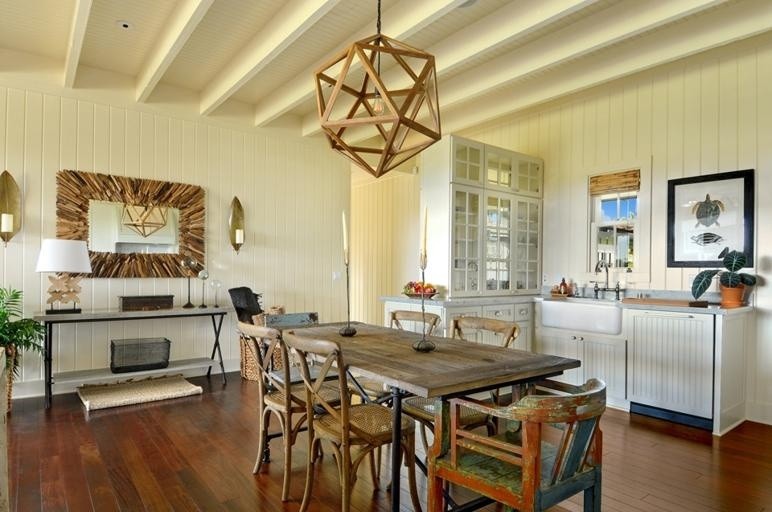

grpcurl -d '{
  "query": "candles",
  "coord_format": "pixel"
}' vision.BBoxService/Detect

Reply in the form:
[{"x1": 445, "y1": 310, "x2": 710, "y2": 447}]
[
  {"x1": 341, "y1": 210, "x2": 349, "y2": 264},
  {"x1": 420, "y1": 202, "x2": 428, "y2": 264}
]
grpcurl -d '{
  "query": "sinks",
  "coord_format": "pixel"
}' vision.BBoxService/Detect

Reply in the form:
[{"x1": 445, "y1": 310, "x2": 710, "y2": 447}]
[{"x1": 533, "y1": 295, "x2": 621, "y2": 336}]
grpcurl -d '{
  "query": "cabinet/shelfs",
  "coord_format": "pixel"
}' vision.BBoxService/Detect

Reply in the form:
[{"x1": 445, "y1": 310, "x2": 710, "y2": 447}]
[
  {"x1": 384, "y1": 303, "x2": 514, "y2": 400},
  {"x1": 515, "y1": 301, "x2": 533, "y2": 351},
  {"x1": 540, "y1": 328, "x2": 628, "y2": 411}
]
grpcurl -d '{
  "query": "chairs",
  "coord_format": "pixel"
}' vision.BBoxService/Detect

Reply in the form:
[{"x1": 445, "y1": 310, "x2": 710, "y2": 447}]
[
  {"x1": 428, "y1": 375, "x2": 607, "y2": 512},
  {"x1": 280, "y1": 327, "x2": 424, "y2": 512},
  {"x1": 340, "y1": 311, "x2": 439, "y2": 478},
  {"x1": 234, "y1": 321, "x2": 352, "y2": 512},
  {"x1": 384, "y1": 318, "x2": 519, "y2": 511}
]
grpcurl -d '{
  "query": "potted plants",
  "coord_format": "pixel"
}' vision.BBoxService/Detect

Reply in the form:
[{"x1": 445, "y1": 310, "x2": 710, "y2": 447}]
[
  {"x1": 691, "y1": 247, "x2": 757, "y2": 308},
  {"x1": 0, "y1": 288, "x2": 52, "y2": 415}
]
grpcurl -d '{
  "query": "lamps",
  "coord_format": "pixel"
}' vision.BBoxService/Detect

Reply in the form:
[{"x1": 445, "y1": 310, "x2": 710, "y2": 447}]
[
  {"x1": 0, "y1": 170, "x2": 22, "y2": 243},
  {"x1": 311, "y1": 0, "x2": 443, "y2": 179},
  {"x1": 34, "y1": 237, "x2": 94, "y2": 314},
  {"x1": 228, "y1": 196, "x2": 244, "y2": 251}
]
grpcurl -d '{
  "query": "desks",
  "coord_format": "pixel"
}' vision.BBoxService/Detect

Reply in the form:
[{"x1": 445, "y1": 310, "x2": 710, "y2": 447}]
[{"x1": 34, "y1": 301, "x2": 235, "y2": 409}]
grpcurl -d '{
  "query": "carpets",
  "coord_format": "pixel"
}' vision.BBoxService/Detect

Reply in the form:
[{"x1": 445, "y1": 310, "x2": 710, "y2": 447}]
[{"x1": 75, "y1": 374, "x2": 203, "y2": 413}]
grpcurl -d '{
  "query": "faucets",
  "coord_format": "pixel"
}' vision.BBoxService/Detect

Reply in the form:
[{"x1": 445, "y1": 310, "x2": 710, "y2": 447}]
[{"x1": 594, "y1": 258, "x2": 609, "y2": 289}]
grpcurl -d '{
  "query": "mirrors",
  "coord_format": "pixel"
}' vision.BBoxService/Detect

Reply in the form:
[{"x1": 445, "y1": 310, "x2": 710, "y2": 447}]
[{"x1": 53, "y1": 167, "x2": 208, "y2": 277}]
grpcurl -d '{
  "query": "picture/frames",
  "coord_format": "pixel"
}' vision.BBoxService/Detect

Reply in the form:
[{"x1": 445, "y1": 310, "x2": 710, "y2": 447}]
[{"x1": 667, "y1": 168, "x2": 754, "y2": 268}]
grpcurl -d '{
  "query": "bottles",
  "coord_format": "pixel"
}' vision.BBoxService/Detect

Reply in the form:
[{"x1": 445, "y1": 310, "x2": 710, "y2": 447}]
[
  {"x1": 553, "y1": 285, "x2": 560, "y2": 293},
  {"x1": 559, "y1": 277, "x2": 567, "y2": 294}
]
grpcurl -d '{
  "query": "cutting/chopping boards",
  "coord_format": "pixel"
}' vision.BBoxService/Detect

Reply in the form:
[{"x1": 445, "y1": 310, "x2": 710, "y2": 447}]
[{"x1": 623, "y1": 297, "x2": 709, "y2": 307}]
